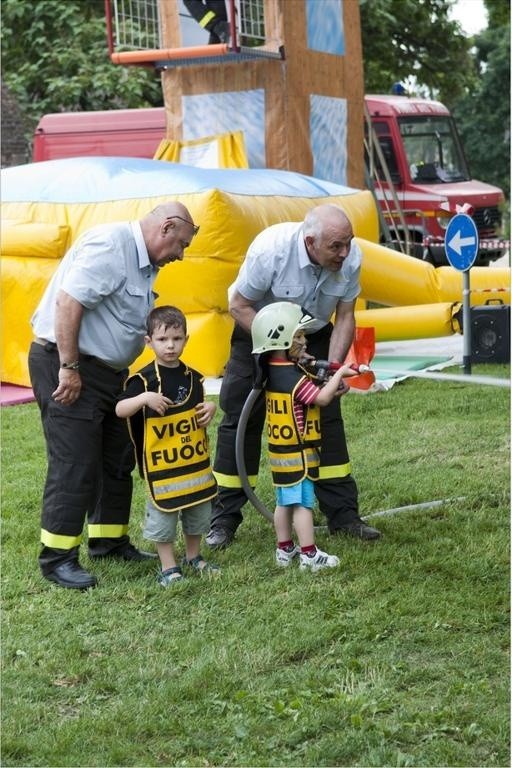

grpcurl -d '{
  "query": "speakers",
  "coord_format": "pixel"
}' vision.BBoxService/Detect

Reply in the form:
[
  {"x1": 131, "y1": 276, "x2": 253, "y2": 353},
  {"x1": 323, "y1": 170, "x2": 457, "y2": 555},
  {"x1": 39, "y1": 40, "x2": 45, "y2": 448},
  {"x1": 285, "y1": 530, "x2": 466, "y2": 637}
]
[{"x1": 470, "y1": 304, "x2": 510, "y2": 363}]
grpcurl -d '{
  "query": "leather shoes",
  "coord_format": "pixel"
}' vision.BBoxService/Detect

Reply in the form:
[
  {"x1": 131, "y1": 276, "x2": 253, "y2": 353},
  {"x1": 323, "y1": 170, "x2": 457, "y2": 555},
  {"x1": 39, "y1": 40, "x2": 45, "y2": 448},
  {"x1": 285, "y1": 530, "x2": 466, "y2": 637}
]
[
  {"x1": 43, "y1": 557, "x2": 96, "y2": 589},
  {"x1": 205, "y1": 521, "x2": 237, "y2": 548},
  {"x1": 327, "y1": 518, "x2": 381, "y2": 541},
  {"x1": 88, "y1": 540, "x2": 160, "y2": 563}
]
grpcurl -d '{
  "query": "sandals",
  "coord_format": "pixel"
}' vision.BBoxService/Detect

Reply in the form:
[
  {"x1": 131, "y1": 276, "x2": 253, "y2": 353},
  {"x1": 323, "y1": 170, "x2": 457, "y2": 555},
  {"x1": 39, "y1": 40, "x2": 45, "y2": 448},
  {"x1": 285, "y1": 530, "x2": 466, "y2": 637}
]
[
  {"x1": 156, "y1": 566, "x2": 188, "y2": 594},
  {"x1": 183, "y1": 555, "x2": 222, "y2": 582}
]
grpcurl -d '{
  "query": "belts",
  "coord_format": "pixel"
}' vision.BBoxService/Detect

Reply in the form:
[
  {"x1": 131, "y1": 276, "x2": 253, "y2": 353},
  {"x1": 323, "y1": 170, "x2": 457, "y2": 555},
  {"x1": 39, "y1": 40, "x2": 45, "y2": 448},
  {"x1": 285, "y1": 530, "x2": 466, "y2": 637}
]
[{"x1": 32, "y1": 336, "x2": 120, "y2": 376}]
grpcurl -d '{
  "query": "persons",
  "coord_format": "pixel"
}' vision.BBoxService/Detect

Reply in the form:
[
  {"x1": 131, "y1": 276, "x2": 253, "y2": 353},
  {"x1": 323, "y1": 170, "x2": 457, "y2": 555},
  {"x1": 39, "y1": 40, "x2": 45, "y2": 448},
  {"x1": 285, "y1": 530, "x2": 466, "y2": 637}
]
[
  {"x1": 28, "y1": 198, "x2": 206, "y2": 590},
  {"x1": 182, "y1": 0, "x2": 234, "y2": 45},
  {"x1": 248, "y1": 301, "x2": 361, "y2": 572},
  {"x1": 111, "y1": 305, "x2": 222, "y2": 589},
  {"x1": 199, "y1": 201, "x2": 384, "y2": 548}
]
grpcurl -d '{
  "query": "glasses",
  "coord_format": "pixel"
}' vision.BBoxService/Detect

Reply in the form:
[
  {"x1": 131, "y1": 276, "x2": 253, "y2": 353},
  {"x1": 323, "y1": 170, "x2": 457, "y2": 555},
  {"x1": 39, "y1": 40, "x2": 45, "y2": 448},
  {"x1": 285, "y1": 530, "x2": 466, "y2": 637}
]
[{"x1": 166, "y1": 214, "x2": 200, "y2": 234}]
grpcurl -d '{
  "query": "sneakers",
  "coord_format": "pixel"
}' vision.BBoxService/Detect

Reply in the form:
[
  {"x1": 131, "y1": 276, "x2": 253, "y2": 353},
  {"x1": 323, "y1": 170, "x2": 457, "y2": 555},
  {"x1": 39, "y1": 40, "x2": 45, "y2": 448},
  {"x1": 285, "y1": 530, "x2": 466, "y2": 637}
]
[
  {"x1": 274, "y1": 540, "x2": 301, "y2": 567},
  {"x1": 296, "y1": 545, "x2": 340, "y2": 572}
]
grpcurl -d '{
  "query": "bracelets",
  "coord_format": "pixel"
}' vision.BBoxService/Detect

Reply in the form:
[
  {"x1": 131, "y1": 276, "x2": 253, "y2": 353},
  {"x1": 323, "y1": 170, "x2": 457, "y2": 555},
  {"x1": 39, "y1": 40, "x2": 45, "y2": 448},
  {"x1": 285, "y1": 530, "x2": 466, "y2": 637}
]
[{"x1": 59, "y1": 361, "x2": 80, "y2": 370}]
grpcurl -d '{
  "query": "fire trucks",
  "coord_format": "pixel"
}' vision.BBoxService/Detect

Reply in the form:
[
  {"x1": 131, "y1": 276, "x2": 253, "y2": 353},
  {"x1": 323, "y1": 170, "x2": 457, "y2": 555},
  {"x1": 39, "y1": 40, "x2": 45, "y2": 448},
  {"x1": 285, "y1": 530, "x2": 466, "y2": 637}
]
[{"x1": 33, "y1": 85, "x2": 505, "y2": 270}]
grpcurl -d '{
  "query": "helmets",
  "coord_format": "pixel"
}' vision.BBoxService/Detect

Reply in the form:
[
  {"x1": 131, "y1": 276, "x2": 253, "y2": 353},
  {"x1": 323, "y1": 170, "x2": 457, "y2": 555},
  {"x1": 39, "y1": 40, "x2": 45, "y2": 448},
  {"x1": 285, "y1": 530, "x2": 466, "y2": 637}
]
[{"x1": 246, "y1": 300, "x2": 316, "y2": 356}]
